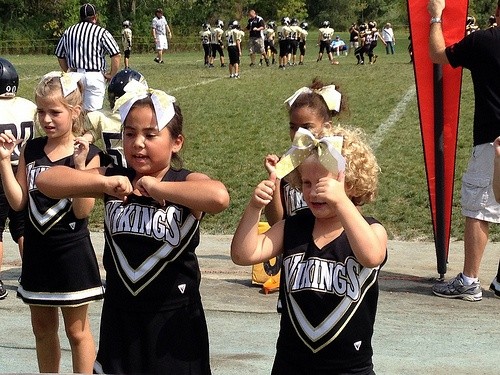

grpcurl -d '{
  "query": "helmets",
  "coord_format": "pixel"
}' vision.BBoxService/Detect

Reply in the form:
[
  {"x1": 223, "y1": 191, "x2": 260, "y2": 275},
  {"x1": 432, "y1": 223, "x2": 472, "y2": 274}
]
[
  {"x1": 107, "y1": 69, "x2": 148, "y2": 110},
  {"x1": 227, "y1": 20, "x2": 241, "y2": 30},
  {"x1": 215, "y1": 19, "x2": 226, "y2": 29},
  {"x1": 122, "y1": 20, "x2": 133, "y2": 28},
  {"x1": 357, "y1": 19, "x2": 378, "y2": 31},
  {"x1": 202, "y1": 23, "x2": 211, "y2": 31},
  {"x1": 281, "y1": 16, "x2": 309, "y2": 31},
  {"x1": 322, "y1": 20, "x2": 331, "y2": 28},
  {"x1": 0, "y1": 57, "x2": 19, "y2": 97},
  {"x1": 267, "y1": 20, "x2": 276, "y2": 28}
]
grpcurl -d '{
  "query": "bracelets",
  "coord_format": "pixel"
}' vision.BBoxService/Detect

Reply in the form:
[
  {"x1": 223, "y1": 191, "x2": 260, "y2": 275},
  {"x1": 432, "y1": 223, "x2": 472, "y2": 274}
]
[{"x1": 430, "y1": 16, "x2": 441, "y2": 23}]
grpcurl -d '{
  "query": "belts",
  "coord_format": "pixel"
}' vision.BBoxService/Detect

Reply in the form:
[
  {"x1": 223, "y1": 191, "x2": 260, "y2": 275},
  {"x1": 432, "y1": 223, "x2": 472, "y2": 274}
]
[{"x1": 70, "y1": 69, "x2": 100, "y2": 72}]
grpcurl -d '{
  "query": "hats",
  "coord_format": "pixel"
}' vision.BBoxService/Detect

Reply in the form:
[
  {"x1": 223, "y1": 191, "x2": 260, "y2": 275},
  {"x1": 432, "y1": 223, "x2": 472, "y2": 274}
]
[{"x1": 80, "y1": 3, "x2": 97, "y2": 17}]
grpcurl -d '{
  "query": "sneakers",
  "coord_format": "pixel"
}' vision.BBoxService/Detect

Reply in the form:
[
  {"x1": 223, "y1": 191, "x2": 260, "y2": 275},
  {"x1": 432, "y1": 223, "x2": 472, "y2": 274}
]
[{"x1": 431, "y1": 273, "x2": 483, "y2": 301}]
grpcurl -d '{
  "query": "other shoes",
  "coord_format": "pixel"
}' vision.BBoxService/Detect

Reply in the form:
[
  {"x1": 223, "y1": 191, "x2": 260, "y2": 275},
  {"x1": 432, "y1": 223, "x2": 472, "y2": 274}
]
[
  {"x1": 204, "y1": 62, "x2": 227, "y2": 68},
  {"x1": 279, "y1": 65, "x2": 286, "y2": 70},
  {"x1": 356, "y1": 60, "x2": 365, "y2": 65},
  {"x1": 287, "y1": 62, "x2": 297, "y2": 66},
  {"x1": 230, "y1": 74, "x2": 241, "y2": 79},
  {"x1": 368, "y1": 55, "x2": 378, "y2": 64},
  {"x1": 299, "y1": 61, "x2": 303, "y2": 65},
  {"x1": 249, "y1": 59, "x2": 276, "y2": 67}
]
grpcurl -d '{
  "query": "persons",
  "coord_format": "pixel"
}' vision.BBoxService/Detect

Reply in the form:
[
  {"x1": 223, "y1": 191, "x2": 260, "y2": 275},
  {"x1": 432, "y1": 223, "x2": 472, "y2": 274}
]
[
  {"x1": 0, "y1": 72, "x2": 103, "y2": 375},
  {"x1": 35, "y1": 80, "x2": 230, "y2": 375},
  {"x1": 0, "y1": 58, "x2": 38, "y2": 298},
  {"x1": 264, "y1": 79, "x2": 348, "y2": 227},
  {"x1": 243, "y1": 10, "x2": 270, "y2": 67},
  {"x1": 121, "y1": 20, "x2": 132, "y2": 69},
  {"x1": 317, "y1": 21, "x2": 412, "y2": 65},
  {"x1": 152, "y1": 9, "x2": 172, "y2": 64},
  {"x1": 84, "y1": 69, "x2": 148, "y2": 284},
  {"x1": 200, "y1": 20, "x2": 244, "y2": 79},
  {"x1": 231, "y1": 125, "x2": 388, "y2": 375},
  {"x1": 55, "y1": 3, "x2": 121, "y2": 112},
  {"x1": 428, "y1": 0, "x2": 500, "y2": 301},
  {"x1": 259, "y1": 17, "x2": 308, "y2": 69}
]
